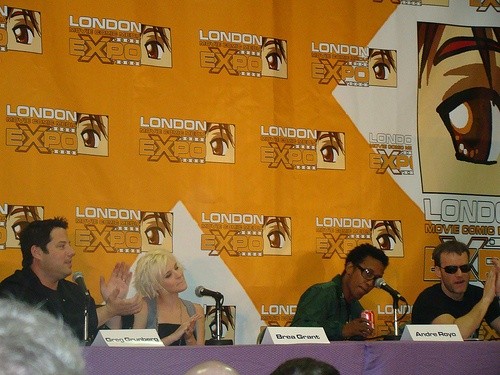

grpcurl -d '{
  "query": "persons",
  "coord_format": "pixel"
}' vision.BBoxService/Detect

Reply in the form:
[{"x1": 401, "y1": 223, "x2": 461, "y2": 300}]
[
  {"x1": 182, "y1": 360, "x2": 241, "y2": 375},
  {"x1": 411, "y1": 241, "x2": 500, "y2": 341},
  {"x1": 0, "y1": 292, "x2": 89, "y2": 374},
  {"x1": 290, "y1": 243, "x2": 390, "y2": 342},
  {"x1": 268, "y1": 358, "x2": 341, "y2": 375},
  {"x1": 0, "y1": 215, "x2": 145, "y2": 348},
  {"x1": 129, "y1": 249, "x2": 207, "y2": 348}
]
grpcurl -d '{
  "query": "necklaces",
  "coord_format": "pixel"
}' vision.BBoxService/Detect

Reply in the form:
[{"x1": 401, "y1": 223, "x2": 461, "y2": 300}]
[{"x1": 156, "y1": 302, "x2": 181, "y2": 333}]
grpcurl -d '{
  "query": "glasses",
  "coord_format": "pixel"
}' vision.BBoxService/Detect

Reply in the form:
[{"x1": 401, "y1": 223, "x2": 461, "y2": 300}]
[
  {"x1": 353, "y1": 262, "x2": 382, "y2": 281},
  {"x1": 439, "y1": 264, "x2": 471, "y2": 274}
]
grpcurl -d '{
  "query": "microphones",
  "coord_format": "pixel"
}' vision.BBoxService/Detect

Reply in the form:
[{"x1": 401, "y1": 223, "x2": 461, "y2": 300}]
[
  {"x1": 195, "y1": 285, "x2": 223, "y2": 300},
  {"x1": 72, "y1": 271, "x2": 91, "y2": 304},
  {"x1": 375, "y1": 278, "x2": 406, "y2": 303}
]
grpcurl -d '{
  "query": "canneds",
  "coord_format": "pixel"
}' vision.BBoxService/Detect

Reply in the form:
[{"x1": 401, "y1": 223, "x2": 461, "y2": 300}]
[{"x1": 361, "y1": 310, "x2": 375, "y2": 331}]
